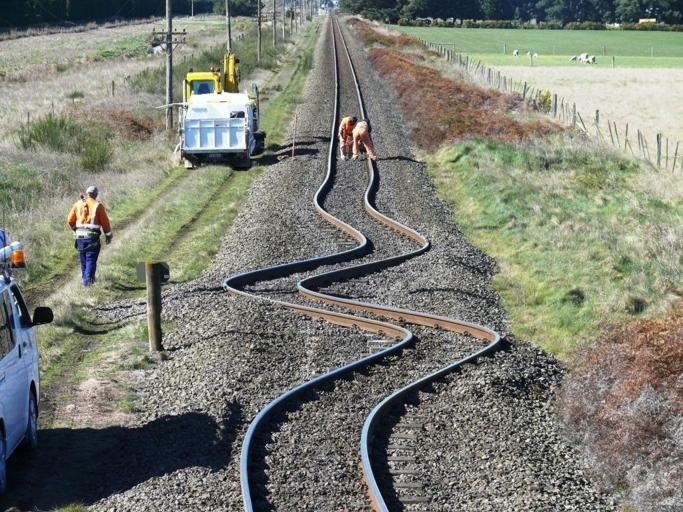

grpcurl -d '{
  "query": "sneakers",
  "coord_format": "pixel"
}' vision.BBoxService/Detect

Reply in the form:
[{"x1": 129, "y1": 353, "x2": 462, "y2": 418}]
[{"x1": 342, "y1": 152, "x2": 379, "y2": 161}]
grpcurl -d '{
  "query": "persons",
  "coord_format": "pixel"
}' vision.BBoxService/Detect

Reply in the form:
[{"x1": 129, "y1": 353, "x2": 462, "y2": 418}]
[
  {"x1": 352, "y1": 119, "x2": 376, "y2": 162},
  {"x1": 337, "y1": 116, "x2": 358, "y2": 160},
  {"x1": 67, "y1": 186, "x2": 112, "y2": 286}
]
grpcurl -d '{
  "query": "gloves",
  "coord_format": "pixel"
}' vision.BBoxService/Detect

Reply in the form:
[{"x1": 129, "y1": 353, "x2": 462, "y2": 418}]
[{"x1": 106, "y1": 236, "x2": 111, "y2": 245}]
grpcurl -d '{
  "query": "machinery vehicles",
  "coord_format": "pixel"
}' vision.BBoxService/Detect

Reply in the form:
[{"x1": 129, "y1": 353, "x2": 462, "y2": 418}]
[{"x1": 174, "y1": 49, "x2": 267, "y2": 174}]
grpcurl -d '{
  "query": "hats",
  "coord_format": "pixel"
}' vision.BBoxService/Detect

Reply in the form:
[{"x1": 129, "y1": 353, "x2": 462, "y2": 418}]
[{"x1": 86, "y1": 185, "x2": 97, "y2": 196}]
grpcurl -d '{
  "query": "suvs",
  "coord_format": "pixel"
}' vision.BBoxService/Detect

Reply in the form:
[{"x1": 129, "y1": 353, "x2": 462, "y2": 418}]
[{"x1": 0, "y1": 226, "x2": 59, "y2": 509}]
